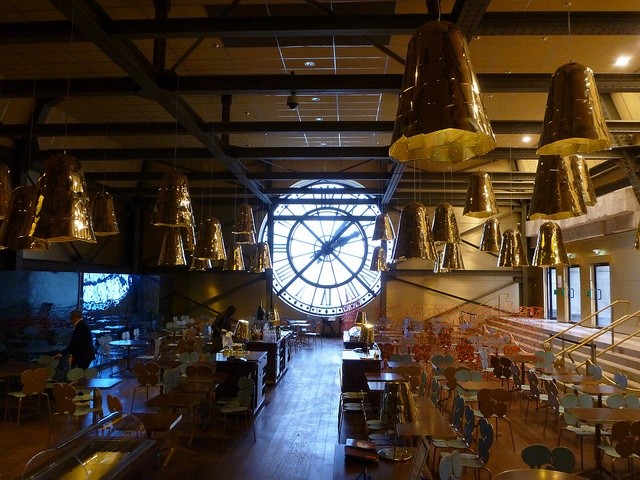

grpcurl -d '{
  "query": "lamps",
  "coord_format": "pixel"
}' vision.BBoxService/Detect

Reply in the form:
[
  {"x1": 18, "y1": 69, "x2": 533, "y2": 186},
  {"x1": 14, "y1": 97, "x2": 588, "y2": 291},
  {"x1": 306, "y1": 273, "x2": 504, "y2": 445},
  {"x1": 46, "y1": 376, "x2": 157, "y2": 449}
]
[
  {"x1": 532, "y1": 215, "x2": 569, "y2": 267},
  {"x1": 18, "y1": 81, "x2": 97, "y2": 243},
  {"x1": 496, "y1": 135, "x2": 530, "y2": 268},
  {"x1": 188, "y1": 190, "x2": 213, "y2": 268},
  {"x1": 433, "y1": 243, "x2": 452, "y2": 273},
  {"x1": 150, "y1": 82, "x2": 195, "y2": 227},
  {"x1": 576, "y1": 156, "x2": 598, "y2": 205},
  {"x1": 157, "y1": 229, "x2": 187, "y2": 266},
  {"x1": 480, "y1": 157, "x2": 502, "y2": 252},
  {"x1": 223, "y1": 237, "x2": 245, "y2": 270},
  {"x1": 535, "y1": 3, "x2": 612, "y2": 156},
  {"x1": 392, "y1": 161, "x2": 432, "y2": 261},
  {"x1": 251, "y1": 199, "x2": 272, "y2": 270},
  {"x1": 378, "y1": 382, "x2": 417, "y2": 462},
  {"x1": 231, "y1": 161, "x2": 257, "y2": 236},
  {"x1": 286, "y1": 93, "x2": 299, "y2": 109},
  {"x1": 463, "y1": 156, "x2": 500, "y2": 217},
  {"x1": 0, "y1": 159, "x2": 13, "y2": 220},
  {"x1": 269, "y1": 308, "x2": 279, "y2": 328},
  {"x1": 358, "y1": 323, "x2": 375, "y2": 360},
  {"x1": 369, "y1": 181, "x2": 387, "y2": 272},
  {"x1": 354, "y1": 311, "x2": 367, "y2": 326},
  {"x1": 91, "y1": 133, "x2": 119, "y2": 236},
  {"x1": 432, "y1": 163, "x2": 460, "y2": 243},
  {"x1": 388, "y1": 2, "x2": 497, "y2": 164},
  {"x1": 527, "y1": 156, "x2": 587, "y2": 219},
  {"x1": 372, "y1": 151, "x2": 395, "y2": 239},
  {"x1": 0, "y1": 85, "x2": 48, "y2": 250},
  {"x1": 443, "y1": 165, "x2": 460, "y2": 270},
  {"x1": 233, "y1": 233, "x2": 255, "y2": 246},
  {"x1": 232, "y1": 320, "x2": 250, "y2": 356},
  {"x1": 191, "y1": 124, "x2": 228, "y2": 260}
]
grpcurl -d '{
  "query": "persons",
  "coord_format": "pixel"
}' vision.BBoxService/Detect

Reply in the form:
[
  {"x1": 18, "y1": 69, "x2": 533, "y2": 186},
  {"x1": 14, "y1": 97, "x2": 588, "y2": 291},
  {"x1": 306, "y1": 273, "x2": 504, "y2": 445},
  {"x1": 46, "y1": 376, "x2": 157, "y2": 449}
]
[
  {"x1": 210, "y1": 305, "x2": 236, "y2": 352},
  {"x1": 54, "y1": 309, "x2": 96, "y2": 369}
]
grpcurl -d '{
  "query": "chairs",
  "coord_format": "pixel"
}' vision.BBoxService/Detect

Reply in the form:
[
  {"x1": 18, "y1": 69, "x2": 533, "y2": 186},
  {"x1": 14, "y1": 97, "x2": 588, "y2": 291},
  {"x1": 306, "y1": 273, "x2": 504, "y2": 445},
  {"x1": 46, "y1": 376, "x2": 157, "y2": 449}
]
[
  {"x1": 130, "y1": 362, "x2": 163, "y2": 414},
  {"x1": 107, "y1": 395, "x2": 121, "y2": 412},
  {"x1": 432, "y1": 356, "x2": 453, "y2": 375},
  {"x1": 521, "y1": 445, "x2": 577, "y2": 473},
  {"x1": 607, "y1": 394, "x2": 639, "y2": 408},
  {"x1": 473, "y1": 389, "x2": 515, "y2": 452},
  {"x1": 433, "y1": 404, "x2": 474, "y2": 472},
  {"x1": 508, "y1": 365, "x2": 531, "y2": 413},
  {"x1": 40, "y1": 356, "x2": 62, "y2": 381},
  {"x1": 418, "y1": 371, "x2": 427, "y2": 398},
  {"x1": 588, "y1": 365, "x2": 602, "y2": 378},
  {"x1": 388, "y1": 354, "x2": 411, "y2": 363},
  {"x1": 122, "y1": 331, "x2": 140, "y2": 351},
  {"x1": 337, "y1": 369, "x2": 372, "y2": 444},
  {"x1": 67, "y1": 368, "x2": 97, "y2": 392},
  {"x1": 3, "y1": 369, "x2": 53, "y2": 428},
  {"x1": 442, "y1": 366, "x2": 470, "y2": 391},
  {"x1": 133, "y1": 328, "x2": 140, "y2": 341},
  {"x1": 615, "y1": 372, "x2": 628, "y2": 389},
  {"x1": 46, "y1": 385, "x2": 99, "y2": 446},
  {"x1": 161, "y1": 370, "x2": 181, "y2": 394},
  {"x1": 542, "y1": 379, "x2": 559, "y2": 434},
  {"x1": 439, "y1": 450, "x2": 464, "y2": 478},
  {"x1": 137, "y1": 339, "x2": 161, "y2": 363},
  {"x1": 597, "y1": 420, "x2": 640, "y2": 480},
  {"x1": 525, "y1": 370, "x2": 549, "y2": 424},
  {"x1": 450, "y1": 396, "x2": 465, "y2": 437},
  {"x1": 221, "y1": 376, "x2": 255, "y2": 446},
  {"x1": 397, "y1": 366, "x2": 421, "y2": 394},
  {"x1": 187, "y1": 365, "x2": 211, "y2": 378},
  {"x1": 490, "y1": 355, "x2": 511, "y2": 392},
  {"x1": 455, "y1": 370, "x2": 482, "y2": 396},
  {"x1": 536, "y1": 350, "x2": 553, "y2": 363},
  {"x1": 431, "y1": 378, "x2": 440, "y2": 407},
  {"x1": 558, "y1": 393, "x2": 611, "y2": 447}
]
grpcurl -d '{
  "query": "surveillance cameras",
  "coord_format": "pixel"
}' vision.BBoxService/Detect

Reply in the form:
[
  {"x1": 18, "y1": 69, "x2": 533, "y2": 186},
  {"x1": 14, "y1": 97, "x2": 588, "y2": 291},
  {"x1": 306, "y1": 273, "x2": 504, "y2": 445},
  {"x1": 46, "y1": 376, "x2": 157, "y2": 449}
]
[{"x1": 287, "y1": 96, "x2": 299, "y2": 110}]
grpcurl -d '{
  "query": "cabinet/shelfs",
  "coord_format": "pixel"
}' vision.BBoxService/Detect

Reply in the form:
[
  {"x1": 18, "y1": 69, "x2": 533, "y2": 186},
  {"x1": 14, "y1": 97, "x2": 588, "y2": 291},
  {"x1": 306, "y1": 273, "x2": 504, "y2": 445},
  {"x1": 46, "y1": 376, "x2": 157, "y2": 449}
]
[
  {"x1": 246, "y1": 330, "x2": 294, "y2": 385},
  {"x1": 215, "y1": 350, "x2": 269, "y2": 412}
]
